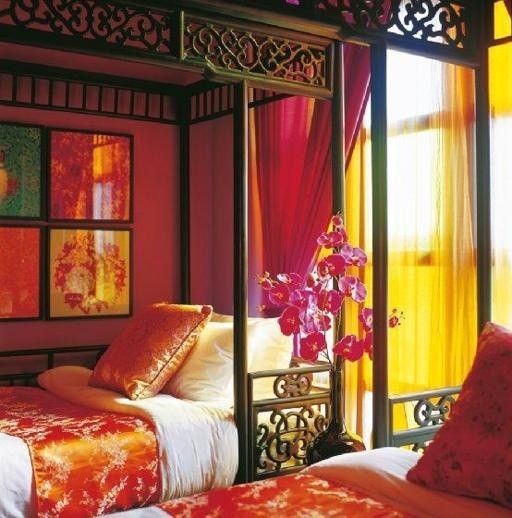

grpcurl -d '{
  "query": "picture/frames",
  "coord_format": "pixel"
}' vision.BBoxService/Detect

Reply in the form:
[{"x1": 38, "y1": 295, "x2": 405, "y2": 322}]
[
  {"x1": 1, "y1": 224, "x2": 49, "y2": 322},
  {"x1": 2, "y1": 121, "x2": 49, "y2": 224},
  {"x1": 44, "y1": 126, "x2": 133, "y2": 224},
  {"x1": 43, "y1": 228, "x2": 135, "y2": 321}
]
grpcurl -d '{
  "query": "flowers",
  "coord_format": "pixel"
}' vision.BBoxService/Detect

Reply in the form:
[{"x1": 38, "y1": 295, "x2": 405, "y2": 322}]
[{"x1": 258, "y1": 215, "x2": 406, "y2": 367}]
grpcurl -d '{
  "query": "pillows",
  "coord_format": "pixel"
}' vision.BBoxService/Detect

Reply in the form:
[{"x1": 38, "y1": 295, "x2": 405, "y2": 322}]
[
  {"x1": 406, "y1": 324, "x2": 511, "y2": 510},
  {"x1": 213, "y1": 311, "x2": 296, "y2": 403},
  {"x1": 89, "y1": 304, "x2": 213, "y2": 399},
  {"x1": 160, "y1": 319, "x2": 261, "y2": 410}
]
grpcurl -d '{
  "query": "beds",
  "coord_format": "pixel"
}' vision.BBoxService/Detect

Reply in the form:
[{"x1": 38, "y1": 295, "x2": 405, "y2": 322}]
[
  {"x1": 3, "y1": 0, "x2": 512, "y2": 517},
  {"x1": 1, "y1": 1, "x2": 351, "y2": 518}
]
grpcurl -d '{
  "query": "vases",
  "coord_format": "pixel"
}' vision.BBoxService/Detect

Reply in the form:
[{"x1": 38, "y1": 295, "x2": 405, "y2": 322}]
[{"x1": 308, "y1": 362, "x2": 367, "y2": 466}]
